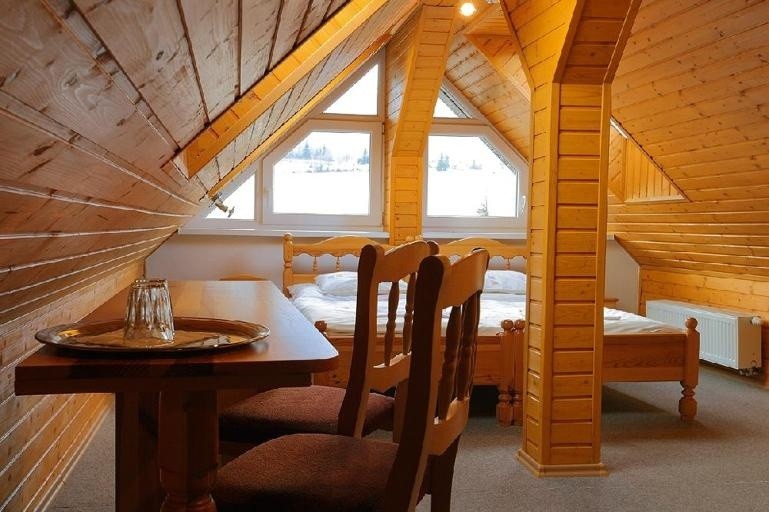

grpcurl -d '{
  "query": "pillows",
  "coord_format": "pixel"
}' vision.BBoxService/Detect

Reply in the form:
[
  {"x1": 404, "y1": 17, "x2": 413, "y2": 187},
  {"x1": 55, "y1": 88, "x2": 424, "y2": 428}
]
[{"x1": 481, "y1": 268, "x2": 529, "y2": 296}]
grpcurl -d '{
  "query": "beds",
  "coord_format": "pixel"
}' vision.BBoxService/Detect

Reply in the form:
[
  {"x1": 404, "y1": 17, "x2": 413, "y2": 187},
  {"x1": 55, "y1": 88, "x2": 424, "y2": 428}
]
[{"x1": 281, "y1": 233, "x2": 703, "y2": 427}]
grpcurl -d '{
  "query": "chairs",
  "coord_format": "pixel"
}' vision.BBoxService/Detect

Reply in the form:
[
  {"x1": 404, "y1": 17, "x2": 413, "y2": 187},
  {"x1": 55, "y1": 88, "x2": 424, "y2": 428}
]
[
  {"x1": 217, "y1": 241, "x2": 443, "y2": 470},
  {"x1": 208, "y1": 253, "x2": 491, "y2": 512}
]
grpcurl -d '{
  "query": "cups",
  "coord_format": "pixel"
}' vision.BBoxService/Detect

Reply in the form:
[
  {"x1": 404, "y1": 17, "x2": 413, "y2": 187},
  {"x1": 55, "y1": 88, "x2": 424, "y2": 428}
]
[{"x1": 121, "y1": 277, "x2": 177, "y2": 348}]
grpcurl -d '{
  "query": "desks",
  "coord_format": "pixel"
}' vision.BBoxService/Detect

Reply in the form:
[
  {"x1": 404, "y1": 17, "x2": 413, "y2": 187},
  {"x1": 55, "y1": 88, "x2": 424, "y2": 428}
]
[{"x1": 13, "y1": 275, "x2": 341, "y2": 512}]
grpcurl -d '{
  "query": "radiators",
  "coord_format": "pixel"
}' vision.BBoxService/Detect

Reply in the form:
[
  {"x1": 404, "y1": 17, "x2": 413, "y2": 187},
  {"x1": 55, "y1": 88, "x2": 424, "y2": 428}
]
[{"x1": 643, "y1": 297, "x2": 764, "y2": 379}]
